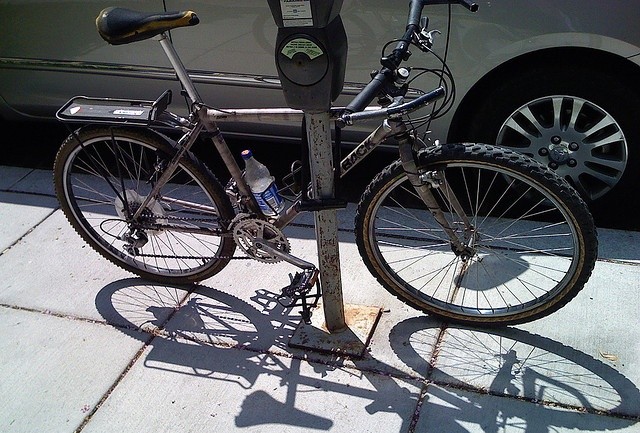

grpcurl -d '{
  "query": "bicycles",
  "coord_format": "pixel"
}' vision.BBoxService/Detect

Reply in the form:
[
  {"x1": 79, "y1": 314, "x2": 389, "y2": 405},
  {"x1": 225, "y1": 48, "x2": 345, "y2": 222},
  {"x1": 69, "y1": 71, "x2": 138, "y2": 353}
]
[{"x1": 52, "y1": 0, "x2": 597, "y2": 329}]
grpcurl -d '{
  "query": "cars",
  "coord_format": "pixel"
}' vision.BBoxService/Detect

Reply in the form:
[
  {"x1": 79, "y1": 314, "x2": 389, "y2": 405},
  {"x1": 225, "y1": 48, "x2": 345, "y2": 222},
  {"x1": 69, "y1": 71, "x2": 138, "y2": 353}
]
[{"x1": 1, "y1": 0, "x2": 640, "y2": 212}]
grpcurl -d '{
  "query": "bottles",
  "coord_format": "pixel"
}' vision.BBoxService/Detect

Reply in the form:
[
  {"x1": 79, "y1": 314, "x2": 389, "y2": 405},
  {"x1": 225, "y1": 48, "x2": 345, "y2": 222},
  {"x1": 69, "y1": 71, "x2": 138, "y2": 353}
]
[{"x1": 242, "y1": 147, "x2": 285, "y2": 215}]
